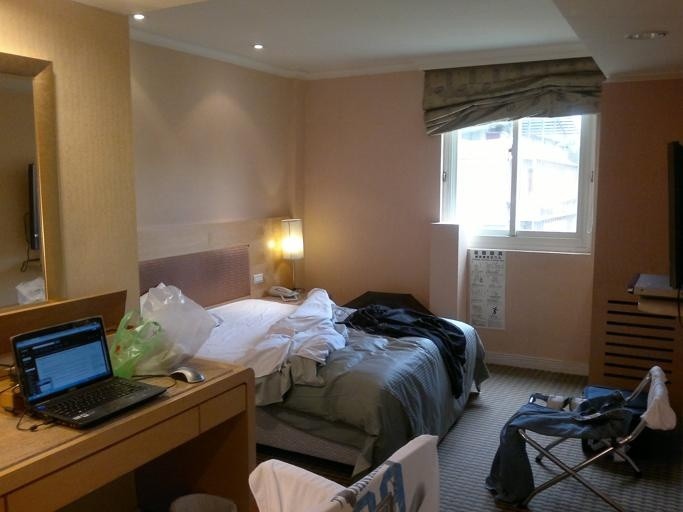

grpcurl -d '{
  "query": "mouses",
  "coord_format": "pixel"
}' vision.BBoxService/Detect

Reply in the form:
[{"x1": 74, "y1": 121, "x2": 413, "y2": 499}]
[{"x1": 169, "y1": 365, "x2": 207, "y2": 384}]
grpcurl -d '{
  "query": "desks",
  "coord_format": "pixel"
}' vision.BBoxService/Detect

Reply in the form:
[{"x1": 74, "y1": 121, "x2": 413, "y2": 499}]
[{"x1": 1, "y1": 331, "x2": 256, "y2": 512}]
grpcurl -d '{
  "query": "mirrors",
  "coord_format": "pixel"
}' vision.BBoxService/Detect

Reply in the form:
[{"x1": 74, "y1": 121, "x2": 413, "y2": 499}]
[{"x1": 0, "y1": 51, "x2": 65, "y2": 316}]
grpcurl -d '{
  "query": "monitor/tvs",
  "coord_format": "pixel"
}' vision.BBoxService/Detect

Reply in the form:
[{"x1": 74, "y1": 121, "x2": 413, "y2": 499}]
[
  {"x1": 666, "y1": 140, "x2": 682, "y2": 289},
  {"x1": 28, "y1": 163, "x2": 39, "y2": 251}
]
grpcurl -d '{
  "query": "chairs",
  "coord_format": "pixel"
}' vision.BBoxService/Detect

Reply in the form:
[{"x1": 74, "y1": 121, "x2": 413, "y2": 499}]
[
  {"x1": 518, "y1": 364, "x2": 667, "y2": 511},
  {"x1": 262, "y1": 433, "x2": 442, "y2": 512}
]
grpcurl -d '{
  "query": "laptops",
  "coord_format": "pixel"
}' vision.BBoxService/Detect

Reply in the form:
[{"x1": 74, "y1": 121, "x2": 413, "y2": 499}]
[{"x1": 10, "y1": 314, "x2": 167, "y2": 430}]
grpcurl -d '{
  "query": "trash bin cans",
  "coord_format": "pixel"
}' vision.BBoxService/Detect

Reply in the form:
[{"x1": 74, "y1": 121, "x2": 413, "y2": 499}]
[{"x1": 171, "y1": 494, "x2": 237, "y2": 511}]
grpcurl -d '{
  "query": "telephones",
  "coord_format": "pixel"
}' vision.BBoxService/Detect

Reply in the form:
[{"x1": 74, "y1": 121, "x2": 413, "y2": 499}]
[{"x1": 267, "y1": 286, "x2": 299, "y2": 297}]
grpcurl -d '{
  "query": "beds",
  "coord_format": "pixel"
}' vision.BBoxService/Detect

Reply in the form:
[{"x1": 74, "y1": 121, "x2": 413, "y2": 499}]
[{"x1": 136, "y1": 244, "x2": 475, "y2": 470}]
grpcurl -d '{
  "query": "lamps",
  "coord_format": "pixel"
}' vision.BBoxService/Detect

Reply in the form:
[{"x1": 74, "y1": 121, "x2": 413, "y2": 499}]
[{"x1": 280, "y1": 218, "x2": 306, "y2": 291}]
